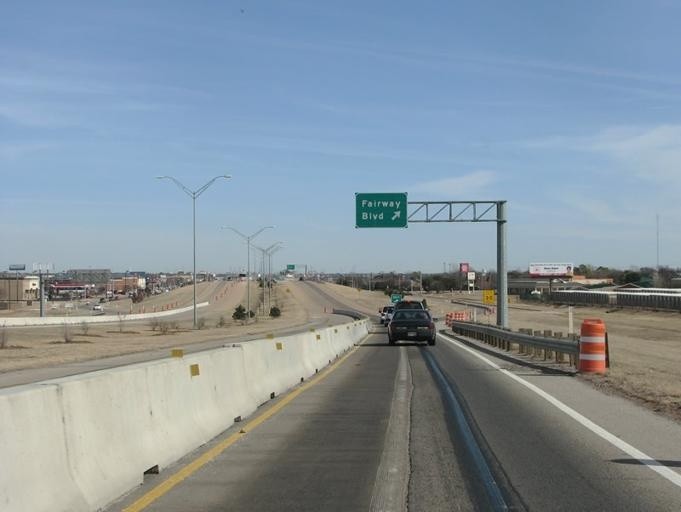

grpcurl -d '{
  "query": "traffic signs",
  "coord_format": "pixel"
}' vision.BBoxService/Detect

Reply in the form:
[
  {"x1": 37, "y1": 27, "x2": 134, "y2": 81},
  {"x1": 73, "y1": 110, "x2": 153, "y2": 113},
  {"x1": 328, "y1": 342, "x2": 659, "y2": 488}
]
[
  {"x1": 391, "y1": 293, "x2": 403, "y2": 303},
  {"x1": 288, "y1": 264, "x2": 295, "y2": 269},
  {"x1": 355, "y1": 192, "x2": 409, "y2": 228}
]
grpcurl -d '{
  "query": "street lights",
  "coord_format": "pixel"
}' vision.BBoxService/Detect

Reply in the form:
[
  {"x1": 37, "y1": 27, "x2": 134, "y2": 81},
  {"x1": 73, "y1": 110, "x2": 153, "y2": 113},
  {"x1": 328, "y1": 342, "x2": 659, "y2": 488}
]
[
  {"x1": 125, "y1": 270, "x2": 129, "y2": 288},
  {"x1": 157, "y1": 173, "x2": 232, "y2": 328},
  {"x1": 219, "y1": 224, "x2": 288, "y2": 317}
]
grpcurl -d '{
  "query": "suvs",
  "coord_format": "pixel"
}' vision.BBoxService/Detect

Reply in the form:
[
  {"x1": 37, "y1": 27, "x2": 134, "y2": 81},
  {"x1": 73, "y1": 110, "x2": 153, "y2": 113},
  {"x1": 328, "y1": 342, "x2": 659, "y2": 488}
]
[
  {"x1": 378, "y1": 306, "x2": 393, "y2": 324},
  {"x1": 392, "y1": 301, "x2": 430, "y2": 316}
]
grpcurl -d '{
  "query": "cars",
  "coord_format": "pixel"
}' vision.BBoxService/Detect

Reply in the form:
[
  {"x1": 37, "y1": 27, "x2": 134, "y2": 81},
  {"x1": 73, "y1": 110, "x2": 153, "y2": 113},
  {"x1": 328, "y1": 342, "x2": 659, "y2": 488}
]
[
  {"x1": 388, "y1": 310, "x2": 438, "y2": 344},
  {"x1": 105, "y1": 286, "x2": 170, "y2": 298},
  {"x1": 93, "y1": 304, "x2": 101, "y2": 310},
  {"x1": 382, "y1": 310, "x2": 393, "y2": 327}
]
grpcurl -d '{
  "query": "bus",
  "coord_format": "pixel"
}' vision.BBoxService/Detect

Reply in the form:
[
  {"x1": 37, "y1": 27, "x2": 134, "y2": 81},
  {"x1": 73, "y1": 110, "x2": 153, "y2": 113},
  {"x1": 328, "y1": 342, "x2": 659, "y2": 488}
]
[{"x1": 51, "y1": 286, "x2": 86, "y2": 297}]
